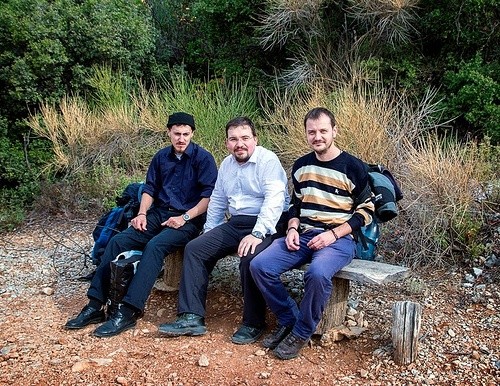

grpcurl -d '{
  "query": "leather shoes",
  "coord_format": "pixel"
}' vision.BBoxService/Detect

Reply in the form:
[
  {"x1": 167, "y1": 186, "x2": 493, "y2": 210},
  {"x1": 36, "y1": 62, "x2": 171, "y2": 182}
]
[
  {"x1": 64, "y1": 305, "x2": 106, "y2": 329},
  {"x1": 92, "y1": 309, "x2": 137, "y2": 336}
]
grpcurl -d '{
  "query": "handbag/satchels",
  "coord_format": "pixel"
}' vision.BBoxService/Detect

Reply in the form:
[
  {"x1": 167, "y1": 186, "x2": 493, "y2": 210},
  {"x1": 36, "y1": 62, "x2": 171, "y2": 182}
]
[{"x1": 107, "y1": 250, "x2": 143, "y2": 320}]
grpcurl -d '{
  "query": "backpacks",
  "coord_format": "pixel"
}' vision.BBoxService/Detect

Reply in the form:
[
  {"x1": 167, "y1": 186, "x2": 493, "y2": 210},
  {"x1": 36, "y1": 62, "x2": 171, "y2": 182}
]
[
  {"x1": 90, "y1": 183, "x2": 144, "y2": 265},
  {"x1": 353, "y1": 164, "x2": 402, "y2": 259}
]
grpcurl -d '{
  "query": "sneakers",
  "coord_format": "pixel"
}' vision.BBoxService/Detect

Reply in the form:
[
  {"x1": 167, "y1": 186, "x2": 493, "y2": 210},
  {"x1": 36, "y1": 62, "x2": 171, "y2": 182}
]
[
  {"x1": 158, "y1": 313, "x2": 206, "y2": 336},
  {"x1": 262, "y1": 325, "x2": 309, "y2": 360},
  {"x1": 232, "y1": 323, "x2": 267, "y2": 345}
]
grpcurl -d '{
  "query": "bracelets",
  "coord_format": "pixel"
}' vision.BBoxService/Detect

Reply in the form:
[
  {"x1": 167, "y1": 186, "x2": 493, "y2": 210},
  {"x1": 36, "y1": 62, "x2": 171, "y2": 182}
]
[
  {"x1": 330, "y1": 229, "x2": 338, "y2": 240},
  {"x1": 137, "y1": 212, "x2": 147, "y2": 216},
  {"x1": 287, "y1": 227, "x2": 297, "y2": 232}
]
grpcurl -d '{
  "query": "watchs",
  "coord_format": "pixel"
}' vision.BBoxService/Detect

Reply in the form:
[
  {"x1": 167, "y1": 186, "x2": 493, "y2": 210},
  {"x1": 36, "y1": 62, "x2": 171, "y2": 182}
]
[
  {"x1": 182, "y1": 213, "x2": 191, "y2": 223},
  {"x1": 251, "y1": 231, "x2": 264, "y2": 240}
]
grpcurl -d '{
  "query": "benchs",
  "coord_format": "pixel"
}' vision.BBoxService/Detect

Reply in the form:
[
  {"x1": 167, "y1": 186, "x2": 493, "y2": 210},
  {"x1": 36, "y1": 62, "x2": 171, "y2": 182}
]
[{"x1": 156, "y1": 248, "x2": 410, "y2": 335}]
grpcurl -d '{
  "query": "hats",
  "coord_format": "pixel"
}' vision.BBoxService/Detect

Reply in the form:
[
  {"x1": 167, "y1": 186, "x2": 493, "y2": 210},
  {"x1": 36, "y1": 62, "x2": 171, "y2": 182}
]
[{"x1": 168, "y1": 112, "x2": 197, "y2": 130}]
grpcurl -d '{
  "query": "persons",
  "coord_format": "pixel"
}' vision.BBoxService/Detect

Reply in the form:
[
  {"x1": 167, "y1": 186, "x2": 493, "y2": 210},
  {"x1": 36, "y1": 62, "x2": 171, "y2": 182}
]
[
  {"x1": 158, "y1": 116, "x2": 290, "y2": 344},
  {"x1": 250, "y1": 107, "x2": 376, "y2": 359},
  {"x1": 64, "y1": 111, "x2": 218, "y2": 337}
]
